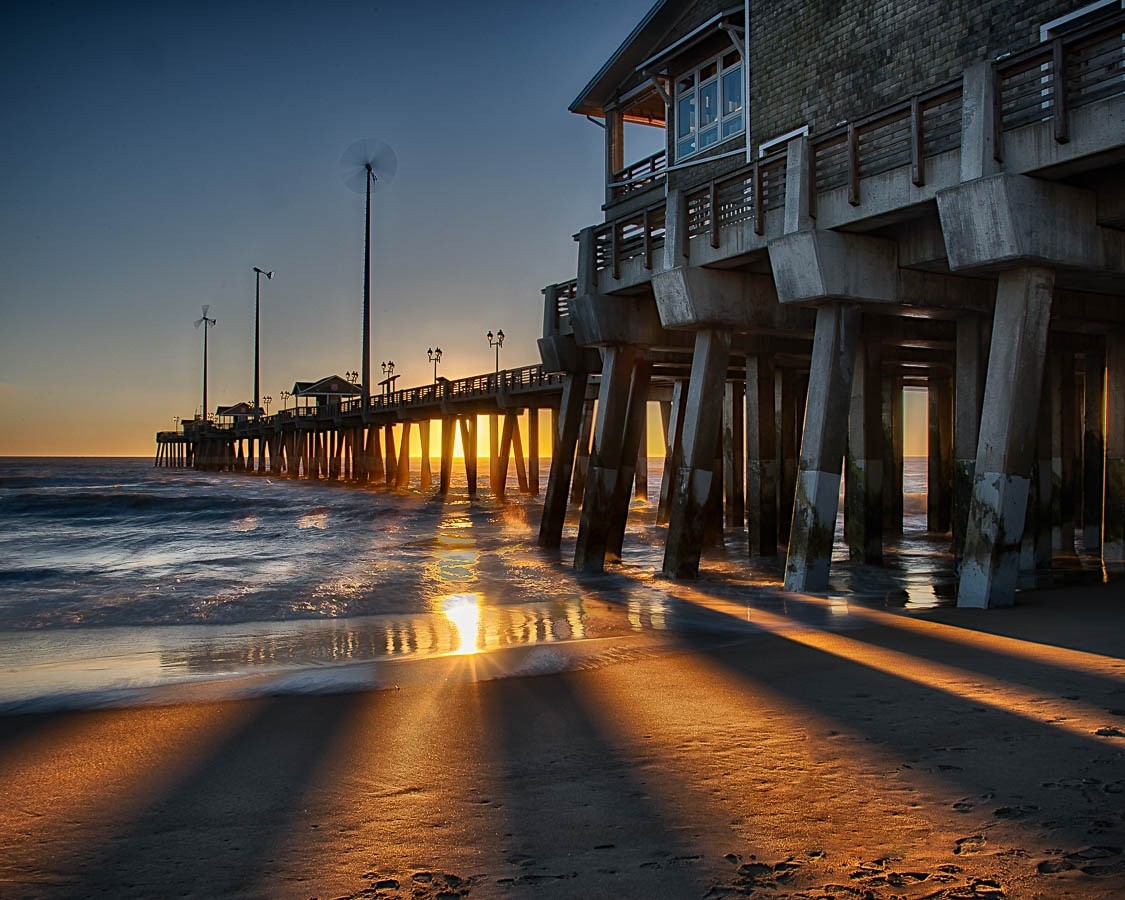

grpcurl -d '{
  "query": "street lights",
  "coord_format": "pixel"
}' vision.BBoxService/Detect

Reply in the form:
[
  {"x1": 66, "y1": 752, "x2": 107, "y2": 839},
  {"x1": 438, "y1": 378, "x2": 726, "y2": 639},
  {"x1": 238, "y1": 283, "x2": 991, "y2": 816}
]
[
  {"x1": 487, "y1": 328, "x2": 505, "y2": 373},
  {"x1": 337, "y1": 136, "x2": 395, "y2": 410},
  {"x1": 262, "y1": 396, "x2": 273, "y2": 418},
  {"x1": 206, "y1": 411, "x2": 215, "y2": 421},
  {"x1": 426, "y1": 347, "x2": 442, "y2": 386},
  {"x1": 380, "y1": 360, "x2": 395, "y2": 405},
  {"x1": 279, "y1": 390, "x2": 291, "y2": 410},
  {"x1": 345, "y1": 370, "x2": 359, "y2": 401},
  {"x1": 253, "y1": 267, "x2": 276, "y2": 424},
  {"x1": 172, "y1": 416, "x2": 181, "y2": 433},
  {"x1": 193, "y1": 304, "x2": 216, "y2": 420}
]
